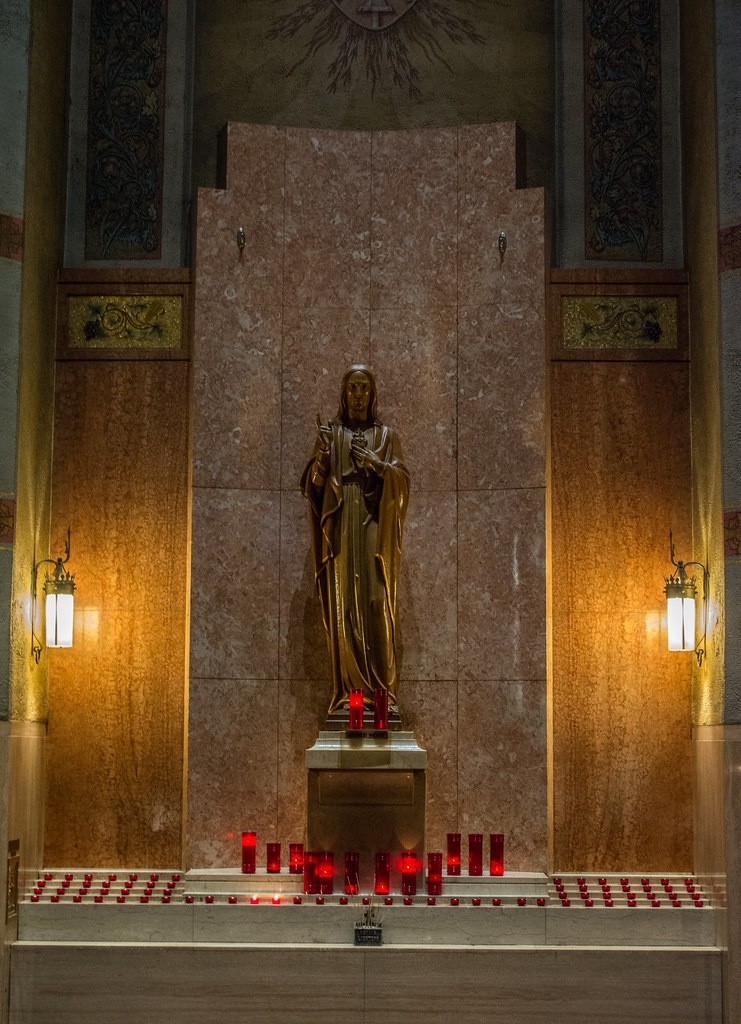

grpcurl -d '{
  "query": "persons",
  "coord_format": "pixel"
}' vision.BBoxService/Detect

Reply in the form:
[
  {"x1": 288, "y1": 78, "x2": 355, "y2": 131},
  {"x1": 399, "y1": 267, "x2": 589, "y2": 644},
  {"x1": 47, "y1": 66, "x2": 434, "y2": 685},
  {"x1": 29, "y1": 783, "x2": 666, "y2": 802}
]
[{"x1": 300, "y1": 364, "x2": 411, "y2": 731}]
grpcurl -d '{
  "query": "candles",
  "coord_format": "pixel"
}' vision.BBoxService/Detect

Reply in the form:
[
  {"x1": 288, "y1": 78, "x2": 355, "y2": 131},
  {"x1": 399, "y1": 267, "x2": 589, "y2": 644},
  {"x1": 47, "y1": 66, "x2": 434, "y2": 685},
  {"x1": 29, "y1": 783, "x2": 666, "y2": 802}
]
[
  {"x1": 553, "y1": 878, "x2": 704, "y2": 907},
  {"x1": 31, "y1": 874, "x2": 180, "y2": 903},
  {"x1": 186, "y1": 896, "x2": 545, "y2": 906}
]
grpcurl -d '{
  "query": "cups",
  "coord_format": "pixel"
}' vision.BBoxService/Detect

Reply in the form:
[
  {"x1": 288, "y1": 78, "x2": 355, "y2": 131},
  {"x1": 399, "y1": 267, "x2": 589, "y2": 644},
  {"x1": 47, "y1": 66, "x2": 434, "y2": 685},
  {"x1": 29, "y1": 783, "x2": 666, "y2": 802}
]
[{"x1": 242, "y1": 687, "x2": 504, "y2": 894}]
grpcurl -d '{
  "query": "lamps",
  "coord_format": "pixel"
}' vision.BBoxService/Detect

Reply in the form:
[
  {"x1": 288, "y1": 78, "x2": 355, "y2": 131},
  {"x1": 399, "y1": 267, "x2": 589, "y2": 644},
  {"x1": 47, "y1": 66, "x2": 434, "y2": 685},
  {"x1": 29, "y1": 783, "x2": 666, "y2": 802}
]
[
  {"x1": 32, "y1": 526, "x2": 77, "y2": 664},
  {"x1": 663, "y1": 529, "x2": 710, "y2": 667}
]
